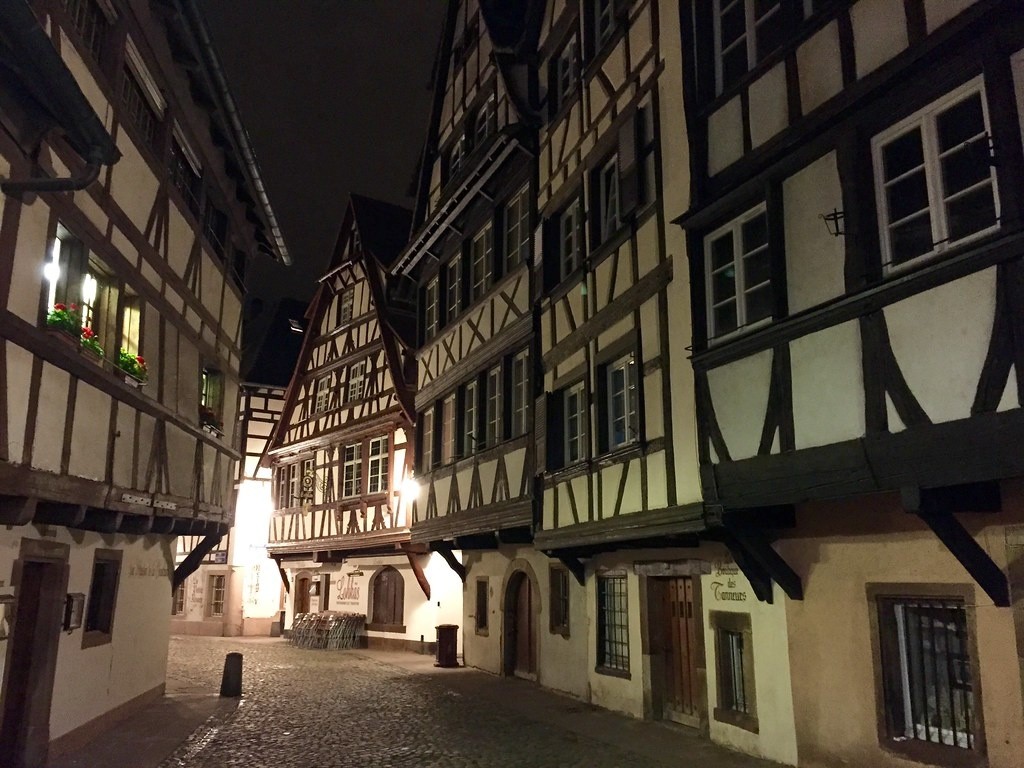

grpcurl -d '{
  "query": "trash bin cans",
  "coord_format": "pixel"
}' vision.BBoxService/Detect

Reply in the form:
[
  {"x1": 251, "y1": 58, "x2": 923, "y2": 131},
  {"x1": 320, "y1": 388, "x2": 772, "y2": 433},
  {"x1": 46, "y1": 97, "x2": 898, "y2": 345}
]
[{"x1": 433, "y1": 625, "x2": 459, "y2": 668}]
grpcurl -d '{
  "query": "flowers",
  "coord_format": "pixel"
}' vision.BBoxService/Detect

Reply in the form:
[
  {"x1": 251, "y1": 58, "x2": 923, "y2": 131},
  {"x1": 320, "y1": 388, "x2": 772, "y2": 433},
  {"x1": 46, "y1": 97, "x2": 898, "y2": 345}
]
[
  {"x1": 118, "y1": 348, "x2": 148, "y2": 380},
  {"x1": 198, "y1": 404, "x2": 224, "y2": 433},
  {"x1": 45, "y1": 302, "x2": 105, "y2": 357}
]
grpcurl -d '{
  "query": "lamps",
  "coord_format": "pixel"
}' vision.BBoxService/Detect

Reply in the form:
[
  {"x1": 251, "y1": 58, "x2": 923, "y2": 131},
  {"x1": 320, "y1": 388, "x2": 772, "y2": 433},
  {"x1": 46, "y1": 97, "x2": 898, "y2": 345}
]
[
  {"x1": 289, "y1": 318, "x2": 303, "y2": 332},
  {"x1": 345, "y1": 565, "x2": 363, "y2": 577}
]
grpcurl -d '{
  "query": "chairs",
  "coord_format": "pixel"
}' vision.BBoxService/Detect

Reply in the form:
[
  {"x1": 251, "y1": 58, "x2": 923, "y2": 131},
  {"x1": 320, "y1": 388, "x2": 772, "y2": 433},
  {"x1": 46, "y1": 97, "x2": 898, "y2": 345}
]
[{"x1": 284, "y1": 611, "x2": 366, "y2": 652}]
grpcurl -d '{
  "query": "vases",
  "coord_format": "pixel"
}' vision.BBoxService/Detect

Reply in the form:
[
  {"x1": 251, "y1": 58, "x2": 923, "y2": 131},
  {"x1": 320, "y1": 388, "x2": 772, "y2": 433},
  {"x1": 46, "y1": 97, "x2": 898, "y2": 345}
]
[
  {"x1": 210, "y1": 427, "x2": 222, "y2": 438},
  {"x1": 76, "y1": 344, "x2": 105, "y2": 368},
  {"x1": 200, "y1": 421, "x2": 214, "y2": 433},
  {"x1": 46, "y1": 324, "x2": 79, "y2": 352},
  {"x1": 113, "y1": 369, "x2": 143, "y2": 387}
]
